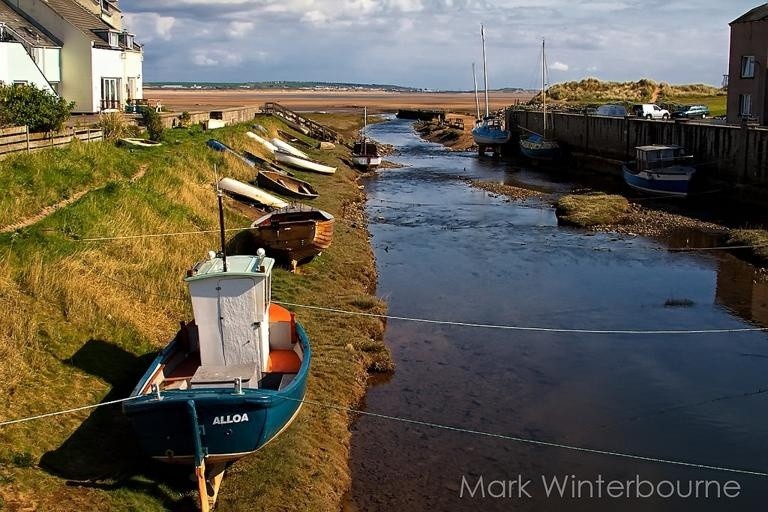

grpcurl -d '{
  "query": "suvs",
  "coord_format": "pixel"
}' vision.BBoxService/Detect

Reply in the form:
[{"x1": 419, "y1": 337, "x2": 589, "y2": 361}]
[{"x1": 632, "y1": 103, "x2": 670, "y2": 120}]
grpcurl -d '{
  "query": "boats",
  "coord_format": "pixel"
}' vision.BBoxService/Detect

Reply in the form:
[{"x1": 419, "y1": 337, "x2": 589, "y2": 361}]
[
  {"x1": 622, "y1": 143, "x2": 696, "y2": 196},
  {"x1": 119, "y1": 300, "x2": 312, "y2": 512},
  {"x1": 353, "y1": 104, "x2": 382, "y2": 172},
  {"x1": 206, "y1": 129, "x2": 338, "y2": 274}
]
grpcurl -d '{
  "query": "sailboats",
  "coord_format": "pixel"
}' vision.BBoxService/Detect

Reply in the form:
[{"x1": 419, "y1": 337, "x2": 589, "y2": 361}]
[
  {"x1": 517, "y1": 38, "x2": 560, "y2": 161},
  {"x1": 471, "y1": 24, "x2": 511, "y2": 157}
]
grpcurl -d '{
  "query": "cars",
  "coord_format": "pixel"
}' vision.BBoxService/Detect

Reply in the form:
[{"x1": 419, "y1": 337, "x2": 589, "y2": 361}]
[
  {"x1": 596, "y1": 105, "x2": 627, "y2": 116},
  {"x1": 671, "y1": 105, "x2": 710, "y2": 119}
]
